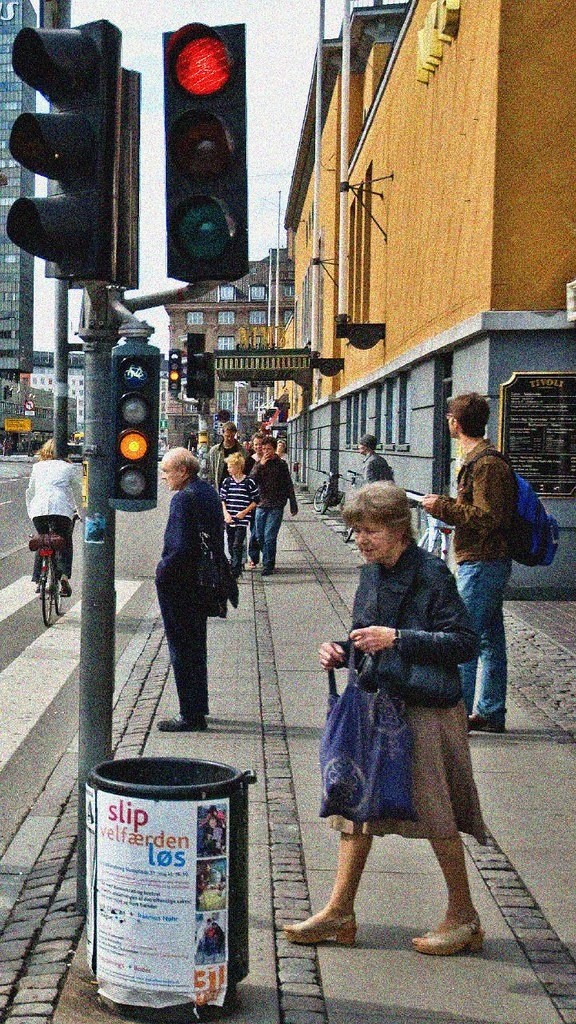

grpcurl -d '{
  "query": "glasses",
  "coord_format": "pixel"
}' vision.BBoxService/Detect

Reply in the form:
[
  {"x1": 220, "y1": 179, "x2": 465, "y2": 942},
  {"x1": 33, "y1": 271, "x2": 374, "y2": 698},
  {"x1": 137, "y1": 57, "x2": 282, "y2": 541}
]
[{"x1": 444, "y1": 413, "x2": 455, "y2": 419}]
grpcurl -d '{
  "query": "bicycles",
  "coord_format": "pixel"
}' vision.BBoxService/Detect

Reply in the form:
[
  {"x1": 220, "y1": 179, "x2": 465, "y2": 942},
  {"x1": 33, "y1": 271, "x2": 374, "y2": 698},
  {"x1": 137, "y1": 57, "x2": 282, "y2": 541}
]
[
  {"x1": 312, "y1": 470, "x2": 340, "y2": 515},
  {"x1": 35, "y1": 512, "x2": 82, "y2": 626},
  {"x1": 342, "y1": 469, "x2": 364, "y2": 543},
  {"x1": 417, "y1": 504, "x2": 452, "y2": 562}
]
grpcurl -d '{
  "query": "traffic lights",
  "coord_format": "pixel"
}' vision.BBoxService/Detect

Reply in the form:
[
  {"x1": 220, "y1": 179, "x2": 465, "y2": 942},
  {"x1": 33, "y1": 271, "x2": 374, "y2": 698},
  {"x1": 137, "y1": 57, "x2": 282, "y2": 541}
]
[
  {"x1": 108, "y1": 343, "x2": 162, "y2": 512},
  {"x1": 4, "y1": 18, "x2": 124, "y2": 282},
  {"x1": 167, "y1": 348, "x2": 182, "y2": 391},
  {"x1": 161, "y1": 22, "x2": 250, "y2": 284}
]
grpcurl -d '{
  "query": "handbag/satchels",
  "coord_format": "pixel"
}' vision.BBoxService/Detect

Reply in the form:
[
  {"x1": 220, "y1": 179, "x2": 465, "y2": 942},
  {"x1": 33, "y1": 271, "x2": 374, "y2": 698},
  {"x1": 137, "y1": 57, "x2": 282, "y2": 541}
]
[
  {"x1": 317, "y1": 641, "x2": 418, "y2": 822},
  {"x1": 198, "y1": 532, "x2": 239, "y2": 618},
  {"x1": 29, "y1": 533, "x2": 63, "y2": 551}
]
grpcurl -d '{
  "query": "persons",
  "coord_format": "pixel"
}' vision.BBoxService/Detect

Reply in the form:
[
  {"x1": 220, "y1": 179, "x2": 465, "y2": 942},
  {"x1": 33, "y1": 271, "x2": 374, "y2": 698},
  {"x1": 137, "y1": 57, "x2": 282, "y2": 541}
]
[
  {"x1": 25, "y1": 438, "x2": 83, "y2": 597},
  {"x1": 208, "y1": 420, "x2": 246, "y2": 494},
  {"x1": 154, "y1": 447, "x2": 222, "y2": 733},
  {"x1": 358, "y1": 434, "x2": 396, "y2": 483},
  {"x1": 220, "y1": 451, "x2": 260, "y2": 581},
  {"x1": 248, "y1": 436, "x2": 298, "y2": 576},
  {"x1": 283, "y1": 480, "x2": 491, "y2": 956},
  {"x1": 237, "y1": 433, "x2": 291, "y2": 568},
  {"x1": 422, "y1": 391, "x2": 512, "y2": 733}
]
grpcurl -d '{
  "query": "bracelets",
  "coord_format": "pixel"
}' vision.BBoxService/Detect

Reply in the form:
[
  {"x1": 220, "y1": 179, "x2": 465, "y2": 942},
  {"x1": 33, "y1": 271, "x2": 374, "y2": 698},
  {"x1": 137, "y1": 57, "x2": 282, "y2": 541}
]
[{"x1": 392, "y1": 628, "x2": 400, "y2": 650}]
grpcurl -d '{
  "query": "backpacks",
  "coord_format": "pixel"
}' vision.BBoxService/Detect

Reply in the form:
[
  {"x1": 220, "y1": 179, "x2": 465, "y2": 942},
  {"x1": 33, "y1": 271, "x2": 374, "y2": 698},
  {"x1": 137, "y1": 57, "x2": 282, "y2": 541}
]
[{"x1": 457, "y1": 449, "x2": 559, "y2": 567}]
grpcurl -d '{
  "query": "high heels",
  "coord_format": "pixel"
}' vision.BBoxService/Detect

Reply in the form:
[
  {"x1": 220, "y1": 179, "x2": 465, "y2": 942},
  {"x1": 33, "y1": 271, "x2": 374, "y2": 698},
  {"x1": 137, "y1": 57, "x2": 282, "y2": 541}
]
[
  {"x1": 412, "y1": 910, "x2": 484, "y2": 955},
  {"x1": 284, "y1": 902, "x2": 356, "y2": 945}
]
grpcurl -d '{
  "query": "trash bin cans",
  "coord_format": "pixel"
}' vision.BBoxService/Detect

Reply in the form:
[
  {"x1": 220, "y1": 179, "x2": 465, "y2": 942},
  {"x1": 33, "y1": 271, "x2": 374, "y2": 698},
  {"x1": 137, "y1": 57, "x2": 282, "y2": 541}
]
[{"x1": 79, "y1": 756, "x2": 258, "y2": 1024}]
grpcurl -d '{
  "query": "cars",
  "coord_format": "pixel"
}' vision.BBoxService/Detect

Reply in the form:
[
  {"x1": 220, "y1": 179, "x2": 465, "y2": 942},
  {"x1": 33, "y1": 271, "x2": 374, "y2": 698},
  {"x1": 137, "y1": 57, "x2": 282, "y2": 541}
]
[{"x1": 157, "y1": 451, "x2": 164, "y2": 461}]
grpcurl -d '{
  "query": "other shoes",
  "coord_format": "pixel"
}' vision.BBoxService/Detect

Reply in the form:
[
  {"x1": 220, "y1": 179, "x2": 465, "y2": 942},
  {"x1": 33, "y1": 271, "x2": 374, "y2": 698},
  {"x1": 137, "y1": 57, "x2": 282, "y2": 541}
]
[
  {"x1": 262, "y1": 567, "x2": 274, "y2": 576},
  {"x1": 468, "y1": 714, "x2": 505, "y2": 734},
  {"x1": 60, "y1": 576, "x2": 72, "y2": 597},
  {"x1": 248, "y1": 560, "x2": 256, "y2": 569},
  {"x1": 35, "y1": 583, "x2": 40, "y2": 592}
]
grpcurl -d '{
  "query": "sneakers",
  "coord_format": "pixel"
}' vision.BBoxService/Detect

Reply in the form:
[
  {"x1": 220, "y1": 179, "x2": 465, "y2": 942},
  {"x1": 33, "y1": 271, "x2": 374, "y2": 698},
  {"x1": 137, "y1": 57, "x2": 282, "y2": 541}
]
[{"x1": 156, "y1": 712, "x2": 207, "y2": 731}]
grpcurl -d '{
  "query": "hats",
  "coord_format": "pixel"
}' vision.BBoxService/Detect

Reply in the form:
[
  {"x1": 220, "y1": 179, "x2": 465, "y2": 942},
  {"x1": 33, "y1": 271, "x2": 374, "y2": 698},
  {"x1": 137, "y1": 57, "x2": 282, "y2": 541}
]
[{"x1": 360, "y1": 434, "x2": 378, "y2": 449}]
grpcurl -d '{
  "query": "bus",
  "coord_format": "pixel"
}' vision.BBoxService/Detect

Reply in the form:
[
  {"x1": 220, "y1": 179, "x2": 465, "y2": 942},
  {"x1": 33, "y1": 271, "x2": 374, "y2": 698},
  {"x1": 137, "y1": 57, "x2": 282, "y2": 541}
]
[{"x1": 66, "y1": 443, "x2": 82, "y2": 462}]
